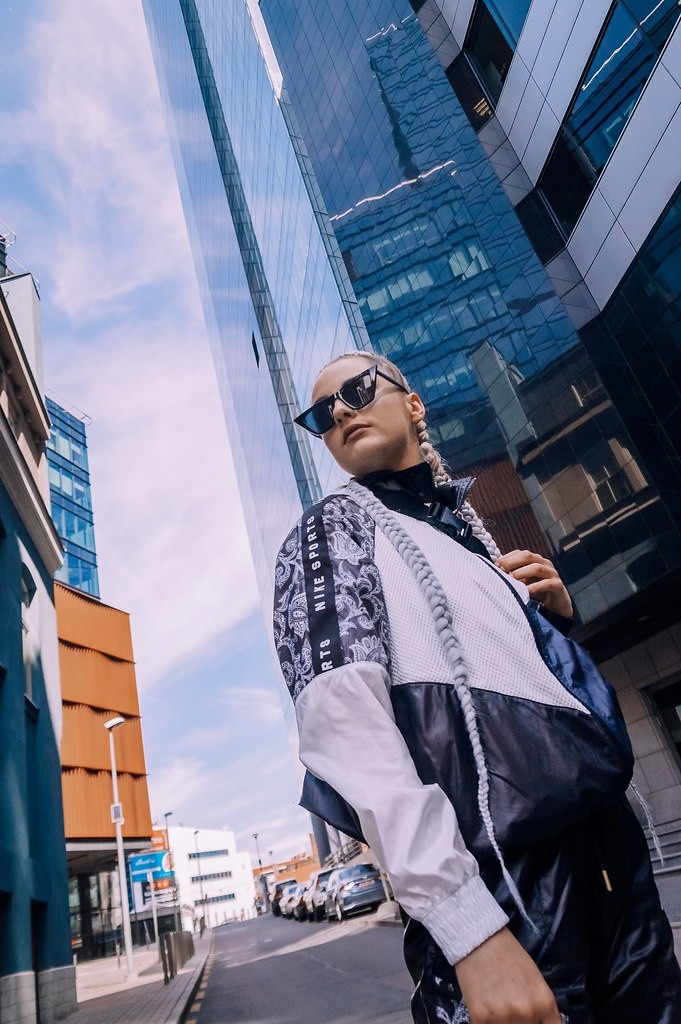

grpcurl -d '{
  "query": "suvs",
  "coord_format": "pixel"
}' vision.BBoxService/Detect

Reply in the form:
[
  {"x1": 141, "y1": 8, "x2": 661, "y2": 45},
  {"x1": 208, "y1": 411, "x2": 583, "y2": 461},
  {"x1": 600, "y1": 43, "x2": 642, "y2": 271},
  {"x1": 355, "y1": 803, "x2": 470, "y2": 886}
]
[{"x1": 269, "y1": 878, "x2": 300, "y2": 916}]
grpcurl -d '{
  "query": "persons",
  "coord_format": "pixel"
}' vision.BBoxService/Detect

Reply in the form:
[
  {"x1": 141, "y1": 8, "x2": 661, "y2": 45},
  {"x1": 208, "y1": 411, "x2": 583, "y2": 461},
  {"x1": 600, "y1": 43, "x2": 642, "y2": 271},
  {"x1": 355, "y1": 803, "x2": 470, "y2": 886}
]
[
  {"x1": 272, "y1": 351, "x2": 681, "y2": 1024},
  {"x1": 254, "y1": 897, "x2": 263, "y2": 917}
]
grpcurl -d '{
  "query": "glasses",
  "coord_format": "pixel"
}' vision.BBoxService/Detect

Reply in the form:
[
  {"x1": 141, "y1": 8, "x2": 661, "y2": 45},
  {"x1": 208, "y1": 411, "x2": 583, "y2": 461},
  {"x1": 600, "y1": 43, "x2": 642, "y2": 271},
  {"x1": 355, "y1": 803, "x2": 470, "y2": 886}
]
[{"x1": 292, "y1": 364, "x2": 409, "y2": 440}]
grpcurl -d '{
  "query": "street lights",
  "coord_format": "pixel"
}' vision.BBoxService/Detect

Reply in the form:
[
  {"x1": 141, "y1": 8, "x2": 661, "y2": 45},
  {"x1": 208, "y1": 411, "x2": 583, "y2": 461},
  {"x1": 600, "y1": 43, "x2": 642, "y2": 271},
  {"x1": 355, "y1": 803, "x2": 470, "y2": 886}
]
[
  {"x1": 191, "y1": 829, "x2": 206, "y2": 937},
  {"x1": 102, "y1": 716, "x2": 137, "y2": 975},
  {"x1": 252, "y1": 833, "x2": 263, "y2": 872},
  {"x1": 164, "y1": 811, "x2": 179, "y2": 931}
]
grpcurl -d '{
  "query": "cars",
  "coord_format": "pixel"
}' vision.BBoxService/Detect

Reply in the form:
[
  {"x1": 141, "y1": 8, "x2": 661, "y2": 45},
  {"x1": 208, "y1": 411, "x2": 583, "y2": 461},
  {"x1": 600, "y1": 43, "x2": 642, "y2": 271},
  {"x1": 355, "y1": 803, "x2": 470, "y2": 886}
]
[{"x1": 278, "y1": 863, "x2": 392, "y2": 923}]
